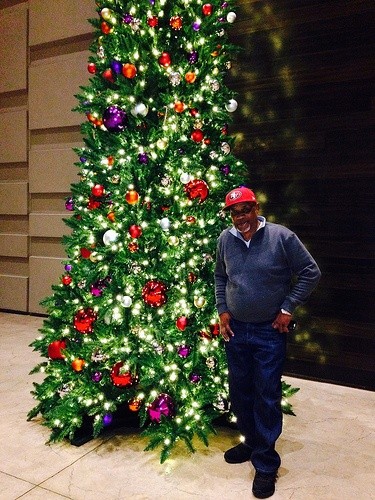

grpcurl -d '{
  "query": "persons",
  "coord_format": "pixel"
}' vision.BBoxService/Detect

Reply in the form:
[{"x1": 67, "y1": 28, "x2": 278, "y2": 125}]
[{"x1": 214, "y1": 186, "x2": 323, "y2": 499}]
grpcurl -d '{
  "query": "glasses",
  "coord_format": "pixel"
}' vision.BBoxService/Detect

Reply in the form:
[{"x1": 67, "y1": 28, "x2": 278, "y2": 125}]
[{"x1": 231, "y1": 202, "x2": 255, "y2": 217}]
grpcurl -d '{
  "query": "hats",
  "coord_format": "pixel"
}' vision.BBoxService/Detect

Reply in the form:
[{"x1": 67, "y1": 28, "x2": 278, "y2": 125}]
[{"x1": 223, "y1": 186, "x2": 256, "y2": 211}]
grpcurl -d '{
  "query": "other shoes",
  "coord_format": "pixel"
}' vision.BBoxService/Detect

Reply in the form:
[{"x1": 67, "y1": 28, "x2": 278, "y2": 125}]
[
  {"x1": 224, "y1": 441, "x2": 251, "y2": 464},
  {"x1": 253, "y1": 470, "x2": 276, "y2": 498}
]
